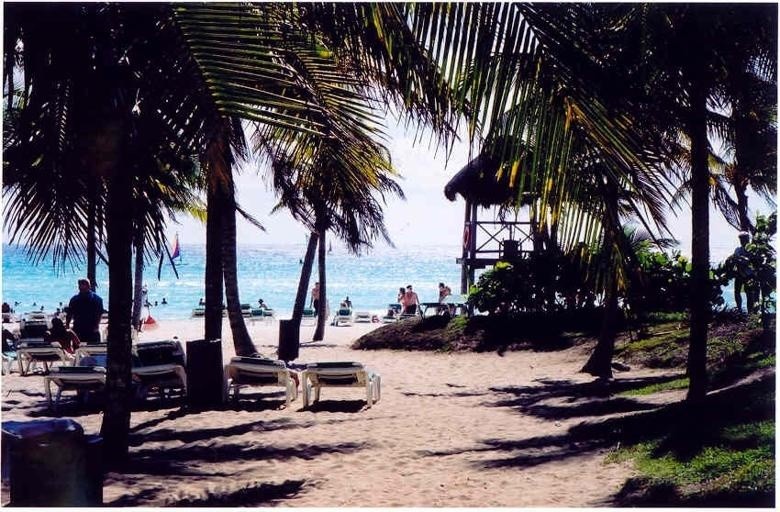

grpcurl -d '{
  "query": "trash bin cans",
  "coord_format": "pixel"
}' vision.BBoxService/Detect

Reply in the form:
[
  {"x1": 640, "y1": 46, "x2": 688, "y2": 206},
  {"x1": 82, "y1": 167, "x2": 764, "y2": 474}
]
[
  {"x1": 278, "y1": 319, "x2": 300, "y2": 367},
  {"x1": 186, "y1": 338, "x2": 224, "y2": 409},
  {"x1": 1, "y1": 419, "x2": 105, "y2": 507}
]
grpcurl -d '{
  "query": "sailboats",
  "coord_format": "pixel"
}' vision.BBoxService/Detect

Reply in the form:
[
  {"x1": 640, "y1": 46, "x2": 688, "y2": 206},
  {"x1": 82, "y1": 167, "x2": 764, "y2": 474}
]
[{"x1": 164, "y1": 233, "x2": 189, "y2": 266}]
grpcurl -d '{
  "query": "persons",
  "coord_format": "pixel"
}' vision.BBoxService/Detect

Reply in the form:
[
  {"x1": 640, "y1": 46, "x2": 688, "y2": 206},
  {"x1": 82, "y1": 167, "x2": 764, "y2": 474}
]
[
  {"x1": 398, "y1": 287, "x2": 405, "y2": 306},
  {"x1": 50, "y1": 317, "x2": 81, "y2": 353},
  {"x1": 435, "y1": 282, "x2": 448, "y2": 316},
  {"x1": 330, "y1": 302, "x2": 348, "y2": 326},
  {"x1": 68, "y1": 276, "x2": 104, "y2": 343},
  {"x1": 733, "y1": 231, "x2": 755, "y2": 314},
  {"x1": 161, "y1": 298, "x2": 167, "y2": 304},
  {"x1": 310, "y1": 281, "x2": 320, "y2": 318},
  {"x1": 258, "y1": 299, "x2": 267, "y2": 309},
  {"x1": 2, "y1": 325, "x2": 14, "y2": 345},
  {"x1": 405, "y1": 285, "x2": 420, "y2": 315},
  {"x1": 2, "y1": 302, "x2": 10, "y2": 323}
]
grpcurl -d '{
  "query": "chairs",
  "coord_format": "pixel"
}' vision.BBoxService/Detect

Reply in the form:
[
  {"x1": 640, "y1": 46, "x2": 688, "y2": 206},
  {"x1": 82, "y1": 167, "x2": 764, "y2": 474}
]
[
  {"x1": 194, "y1": 305, "x2": 416, "y2": 327},
  {"x1": 4, "y1": 303, "x2": 109, "y2": 408},
  {"x1": 227, "y1": 357, "x2": 299, "y2": 408},
  {"x1": 301, "y1": 363, "x2": 381, "y2": 408},
  {"x1": 134, "y1": 341, "x2": 186, "y2": 403}
]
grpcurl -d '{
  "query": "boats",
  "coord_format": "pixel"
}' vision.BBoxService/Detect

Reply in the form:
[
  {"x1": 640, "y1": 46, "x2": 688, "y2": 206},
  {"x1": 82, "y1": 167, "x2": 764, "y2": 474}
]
[{"x1": 192, "y1": 303, "x2": 274, "y2": 317}]
